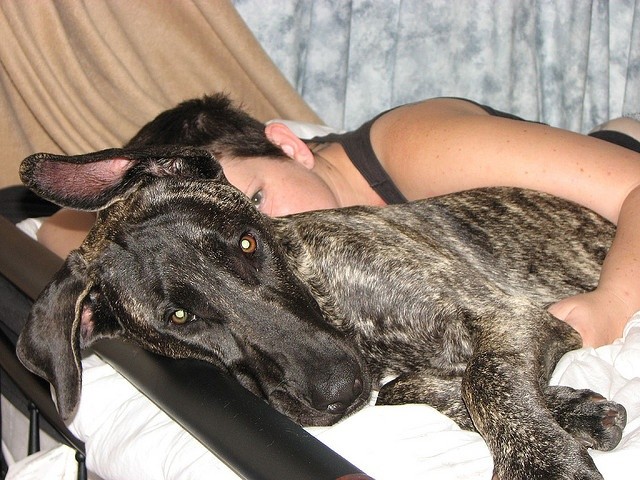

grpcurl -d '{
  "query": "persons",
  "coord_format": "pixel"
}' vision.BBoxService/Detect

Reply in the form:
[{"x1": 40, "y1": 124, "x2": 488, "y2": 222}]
[{"x1": 122, "y1": 92, "x2": 640, "y2": 349}]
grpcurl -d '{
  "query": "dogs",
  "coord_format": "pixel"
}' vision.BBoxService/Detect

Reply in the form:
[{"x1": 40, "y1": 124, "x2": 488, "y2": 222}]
[{"x1": 13, "y1": 148, "x2": 627, "y2": 480}]
